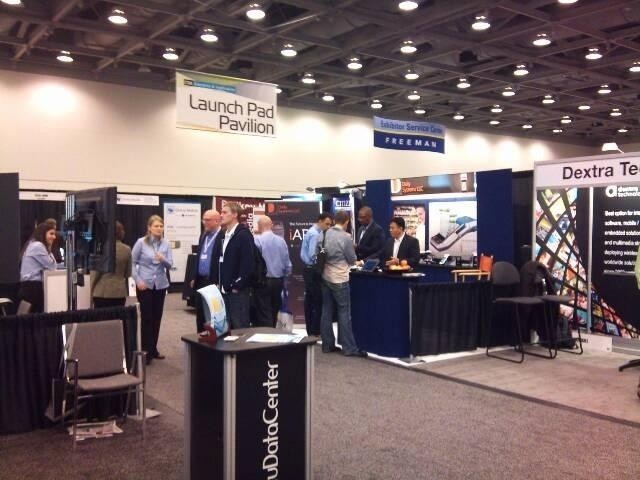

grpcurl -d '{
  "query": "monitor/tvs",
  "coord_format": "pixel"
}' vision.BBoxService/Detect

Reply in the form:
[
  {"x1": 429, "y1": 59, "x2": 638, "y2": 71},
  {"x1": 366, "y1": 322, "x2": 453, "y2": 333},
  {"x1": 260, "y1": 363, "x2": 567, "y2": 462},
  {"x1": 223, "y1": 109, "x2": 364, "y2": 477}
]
[{"x1": 66, "y1": 186, "x2": 116, "y2": 274}]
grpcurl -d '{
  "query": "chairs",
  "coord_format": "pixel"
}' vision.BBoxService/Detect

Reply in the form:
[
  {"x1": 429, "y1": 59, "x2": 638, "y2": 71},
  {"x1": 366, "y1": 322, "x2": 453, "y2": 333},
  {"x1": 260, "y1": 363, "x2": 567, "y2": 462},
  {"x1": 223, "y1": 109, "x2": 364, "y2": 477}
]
[
  {"x1": 450, "y1": 253, "x2": 584, "y2": 364},
  {"x1": 51, "y1": 319, "x2": 148, "y2": 453}
]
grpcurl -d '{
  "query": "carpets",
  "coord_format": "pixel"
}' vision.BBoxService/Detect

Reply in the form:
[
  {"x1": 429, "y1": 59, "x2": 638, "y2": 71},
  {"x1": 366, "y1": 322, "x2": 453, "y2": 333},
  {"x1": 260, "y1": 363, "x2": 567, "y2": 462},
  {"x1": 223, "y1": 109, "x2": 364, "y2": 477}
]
[{"x1": 293, "y1": 322, "x2": 640, "y2": 428}]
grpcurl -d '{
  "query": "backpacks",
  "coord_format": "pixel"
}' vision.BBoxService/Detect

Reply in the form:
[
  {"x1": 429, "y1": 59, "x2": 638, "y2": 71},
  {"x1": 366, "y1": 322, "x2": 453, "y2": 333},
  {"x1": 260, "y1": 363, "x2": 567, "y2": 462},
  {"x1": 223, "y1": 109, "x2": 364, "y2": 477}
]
[{"x1": 242, "y1": 228, "x2": 267, "y2": 289}]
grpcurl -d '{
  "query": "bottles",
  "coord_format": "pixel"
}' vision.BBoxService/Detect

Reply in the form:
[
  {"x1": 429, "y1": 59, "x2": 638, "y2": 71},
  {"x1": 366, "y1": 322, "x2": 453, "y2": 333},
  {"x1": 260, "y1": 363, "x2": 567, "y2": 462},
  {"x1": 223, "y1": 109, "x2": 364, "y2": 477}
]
[{"x1": 277, "y1": 278, "x2": 293, "y2": 333}]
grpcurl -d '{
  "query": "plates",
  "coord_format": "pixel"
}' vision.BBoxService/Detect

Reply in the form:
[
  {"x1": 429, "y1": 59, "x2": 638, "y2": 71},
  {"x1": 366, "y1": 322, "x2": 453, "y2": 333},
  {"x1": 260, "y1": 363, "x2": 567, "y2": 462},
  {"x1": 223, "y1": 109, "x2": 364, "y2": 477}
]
[
  {"x1": 402, "y1": 272, "x2": 427, "y2": 278},
  {"x1": 388, "y1": 268, "x2": 413, "y2": 273}
]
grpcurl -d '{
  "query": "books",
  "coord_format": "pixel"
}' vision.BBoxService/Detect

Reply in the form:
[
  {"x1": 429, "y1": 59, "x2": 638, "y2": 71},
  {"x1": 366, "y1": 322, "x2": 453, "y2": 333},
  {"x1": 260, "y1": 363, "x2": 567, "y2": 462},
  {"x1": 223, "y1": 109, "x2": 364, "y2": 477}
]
[{"x1": 67, "y1": 419, "x2": 125, "y2": 442}]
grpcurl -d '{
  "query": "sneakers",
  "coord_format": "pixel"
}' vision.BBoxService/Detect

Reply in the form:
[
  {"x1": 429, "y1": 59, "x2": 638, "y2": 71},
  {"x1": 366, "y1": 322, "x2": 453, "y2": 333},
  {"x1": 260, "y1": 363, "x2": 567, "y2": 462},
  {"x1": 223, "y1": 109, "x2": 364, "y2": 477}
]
[
  {"x1": 322, "y1": 347, "x2": 341, "y2": 352},
  {"x1": 344, "y1": 348, "x2": 366, "y2": 356},
  {"x1": 155, "y1": 353, "x2": 165, "y2": 359}
]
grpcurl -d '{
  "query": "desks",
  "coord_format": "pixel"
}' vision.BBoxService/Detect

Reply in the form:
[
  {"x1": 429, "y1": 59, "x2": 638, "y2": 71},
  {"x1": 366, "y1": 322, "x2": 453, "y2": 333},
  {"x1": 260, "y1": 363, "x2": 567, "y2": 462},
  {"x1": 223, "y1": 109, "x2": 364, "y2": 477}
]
[{"x1": 349, "y1": 270, "x2": 491, "y2": 364}]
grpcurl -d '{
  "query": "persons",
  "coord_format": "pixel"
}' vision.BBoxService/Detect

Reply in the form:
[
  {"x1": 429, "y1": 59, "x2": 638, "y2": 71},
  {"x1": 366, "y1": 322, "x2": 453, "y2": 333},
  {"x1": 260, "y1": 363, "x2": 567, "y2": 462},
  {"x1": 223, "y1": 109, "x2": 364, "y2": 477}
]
[
  {"x1": 131, "y1": 214, "x2": 173, "y2": 366},
  {"x1": 357, "y1": 212, "x2": 421, "y2": 272},
  {"x1": 190, "y1": 208, "x2": 229, "y2": 333},
  {"x1": 44, "y1": 217, "x2": 66, "y2": 263},
  {"x1": 416, "y1": 207, "x2": 426, "y2": 252},
  {"x1": 214, "y1": 203, "x2": 258, "y2": 328},
  {"x1": 16, "y1": 222, "x2": 58, "y2": 313},
  {"x1": 90, "y1": 220, "x2": 132, "y2": 309},
  {"x1": 253, "y1": 217, "x2": 293, "y2": 329},
  {"x1": 355, "y1": 207, "x2": 384, "y2": 263},
  {"x1": 320, "y1": 211, "x2": 368, "y2": 356},
  {"x1": 300, "y1": 214, "x2": 331, "y2": 333}
]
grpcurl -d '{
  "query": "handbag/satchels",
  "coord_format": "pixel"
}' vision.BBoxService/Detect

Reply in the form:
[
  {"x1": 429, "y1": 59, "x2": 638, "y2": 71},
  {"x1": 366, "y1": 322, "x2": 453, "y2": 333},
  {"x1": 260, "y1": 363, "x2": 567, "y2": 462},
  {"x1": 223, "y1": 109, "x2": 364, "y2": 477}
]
[
  {"x1": 42, "y1": 365, "x2": 65, "y2": 396},
  {"x1": 313, "y1": 248, "x2": 326, "y2": 277},
  {"x1": 276, "y1": 310, "x2": 293, "y2": 333}
]
